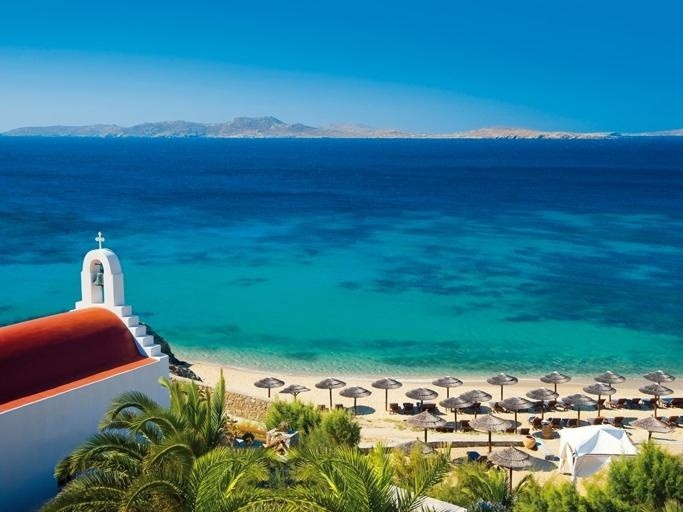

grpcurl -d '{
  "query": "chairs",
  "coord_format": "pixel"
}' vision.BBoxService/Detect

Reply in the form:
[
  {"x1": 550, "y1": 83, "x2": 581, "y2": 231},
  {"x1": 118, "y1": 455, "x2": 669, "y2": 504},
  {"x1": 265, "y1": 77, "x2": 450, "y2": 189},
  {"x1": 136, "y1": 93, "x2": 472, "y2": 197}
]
[{"x1": 388, "y1": 396, "x2": 682, "y2": 434}]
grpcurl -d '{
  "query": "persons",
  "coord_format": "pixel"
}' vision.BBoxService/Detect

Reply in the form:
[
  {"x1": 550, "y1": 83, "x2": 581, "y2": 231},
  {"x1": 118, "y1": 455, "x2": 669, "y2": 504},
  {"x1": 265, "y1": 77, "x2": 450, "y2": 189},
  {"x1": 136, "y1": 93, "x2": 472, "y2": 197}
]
[{"x1": 239, "y1": 432, "x2": 265, "y2": 448}]
